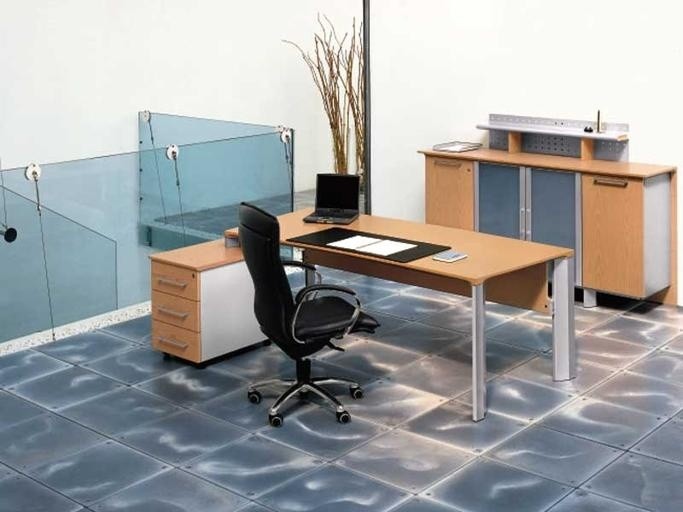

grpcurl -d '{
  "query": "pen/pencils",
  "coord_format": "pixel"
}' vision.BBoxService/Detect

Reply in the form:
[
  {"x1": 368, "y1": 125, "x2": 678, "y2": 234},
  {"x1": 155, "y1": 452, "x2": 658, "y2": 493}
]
[{"x1": 598, "y1": 110, "x2": 600, "y2": 133}]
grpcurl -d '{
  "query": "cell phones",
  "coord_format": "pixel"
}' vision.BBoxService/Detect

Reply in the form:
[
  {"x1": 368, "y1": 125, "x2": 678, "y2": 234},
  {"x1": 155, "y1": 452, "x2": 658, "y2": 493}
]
[{"x1": 432, "y1": 251, "x2": 468, "y2": 263}]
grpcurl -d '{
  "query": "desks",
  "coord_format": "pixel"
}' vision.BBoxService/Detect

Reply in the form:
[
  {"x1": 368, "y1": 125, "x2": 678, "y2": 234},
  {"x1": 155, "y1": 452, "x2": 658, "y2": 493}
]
[{"x1": 226, "y1": 207, "x2": 573, "y2": 421}]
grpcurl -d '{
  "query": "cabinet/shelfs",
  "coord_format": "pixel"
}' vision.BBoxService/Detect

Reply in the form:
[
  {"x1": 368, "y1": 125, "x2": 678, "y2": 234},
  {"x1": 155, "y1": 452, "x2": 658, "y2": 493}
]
[
  {"x1": 146, "y1": 237, "x2": 271, "y2": 371},
  {"x1": 417, "y1": 141, "x2": 676, "y2": 316}
]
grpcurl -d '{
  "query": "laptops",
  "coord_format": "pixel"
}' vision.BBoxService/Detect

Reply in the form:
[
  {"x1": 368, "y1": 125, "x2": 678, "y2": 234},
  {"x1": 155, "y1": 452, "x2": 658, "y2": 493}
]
[{"x1": 303, "y1": 174, "x2": 360, "y2": 225}]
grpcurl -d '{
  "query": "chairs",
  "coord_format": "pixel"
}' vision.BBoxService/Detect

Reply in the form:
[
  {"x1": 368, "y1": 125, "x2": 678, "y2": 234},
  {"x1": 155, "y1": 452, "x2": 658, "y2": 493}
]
[{"x1": 237, "y1": 202, "x2": 382, "y2": 426}]
